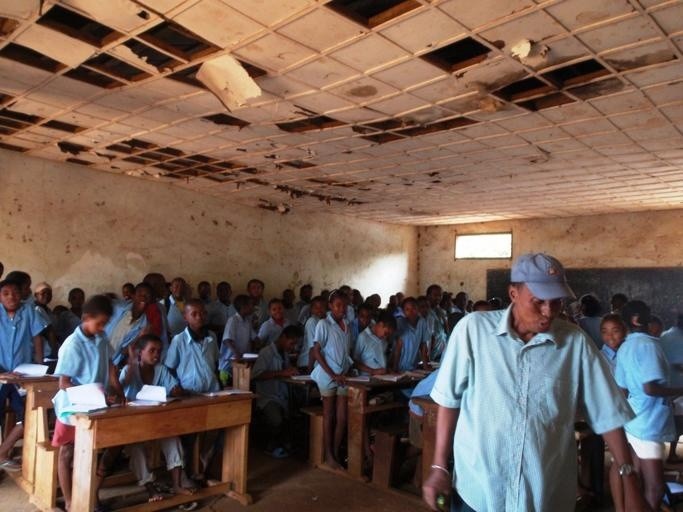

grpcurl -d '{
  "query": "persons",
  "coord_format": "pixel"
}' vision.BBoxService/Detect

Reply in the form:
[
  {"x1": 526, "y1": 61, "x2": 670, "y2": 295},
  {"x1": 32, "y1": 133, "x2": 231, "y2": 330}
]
[
  {"x1": 297, "y1": 284, "x2": 321, "y2": 318},
  {"x1": 600, "y1": 315, "x2": 626, "y2": 365},
  {"x1": 352, "y1": 314, "x2": 397, "y2": 375},
  {"x1": 158, "y1": 278, "x2": 197, "y2": 340},
  {"x1": 257, "y1": 298, "x2": 297, "y2": 349},
  {"x1": 218, "y1": 294, "x2": 258, "y2": 387},
  {"x1": 421, "y1": 254, "x2": 651, "y2": 512},
  {"x1": 601, "y1": 293, "x2": 629, "y2": 321},
  {"x1": 281, "y1": 288, "x2": 299, "y2": 320},
  {"x1": 58, "y1": 288, "x2": 89, "y2": 343},
  {"x1": 473, "y1": 300, "x2": 490, "y2": 311},
  {"x1": 105, "y1": 282, "x2": 148, "y2": 366},
  {"x1": 248, "y1": 279, "x2": 267, "y2": 305},
  {"x1": 575, "y1": 295, "x2": 609, "y2": 347},
  {"x1": 311, "y1": 290, "x2": 352, "y2": 472},
  {"x1": 191, "y1": 282, "x2": 219, "y2": 320},
  {"x1": 298, "y1": 296, "x2": 329, "y2": 374},
  {"x1": 321, "y1": 286, "x2": 417, "y2": 309},
  {"x1": 615, "y1": 299, "x2": 683, "y2": 512},
  {"x1": 142, "y1": 272, "x2": 167, "y2": 355},
  {"x1": 209, "y1": 283, "x2": 240, "y2": 325},
  {"x1": 35, "y1": 283, "x2": 52, "y2": 310},
  {"x1": 122, "y1": 284, "x2": 135, "y2": 301},
  {"x1": 5, "y1": 271, "x2": 32, "y2": 308},
  {"x1": 393, "y1": 296, "x2": 433, "y2": 372},
  {"x1": 426, "y1": 284, "x2": 450, "y2": 333},
  {"x1": 51, "y1": 294, "x2": 126, "y2": 512},
  {"x1": 349, "y1": 303, "x2": 380, "y2": 351},
  {"x1": 418, "y1": 296, "x2": 432, "y2": 359},
  {"x1": 164, "y1": 299, "x2": 220, "y2": 391},
  {"x1": 0, "y1": 281, "x2": 50, "y2": 472},
  {"x1": 447, "y1": 291, "x2": 470, "y2": 315},
  {"x1": 660, "y1": 313, "x2": 683, "y2": 396},
  {"x1": 253, "y1": 325, "x2": 301, "y2": 458},
  {"x1": 648, "y1": 316, "x2": 662, "y2": 336},
  {"x1": 118, "y1": 334, "x2": 198, "y2": 501}
]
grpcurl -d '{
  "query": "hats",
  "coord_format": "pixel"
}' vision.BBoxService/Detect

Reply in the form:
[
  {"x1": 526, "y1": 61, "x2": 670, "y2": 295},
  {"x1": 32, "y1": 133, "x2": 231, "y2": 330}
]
[
  {"x1": 35, "y1": 283, "x2": 52, "y2": 293},
  {"x1": 510, "y1": 253, "x2": 577, "y2": 300}
]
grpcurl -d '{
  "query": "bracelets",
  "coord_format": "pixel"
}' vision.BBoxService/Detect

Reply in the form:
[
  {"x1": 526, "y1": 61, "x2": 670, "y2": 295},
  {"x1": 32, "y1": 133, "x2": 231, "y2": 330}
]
[{"x1": 431, "y1": 464, "x2": 449, "y2": 477}]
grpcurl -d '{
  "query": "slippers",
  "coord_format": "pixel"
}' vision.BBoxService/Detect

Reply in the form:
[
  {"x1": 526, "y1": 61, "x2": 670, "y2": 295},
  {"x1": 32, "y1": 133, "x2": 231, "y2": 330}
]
[
  {"x1": 0, "y1": 460, "x2": 22, "y2": 472},
  {"x1": 264, "y1": 447, "x2": 287, "y2": 459}
]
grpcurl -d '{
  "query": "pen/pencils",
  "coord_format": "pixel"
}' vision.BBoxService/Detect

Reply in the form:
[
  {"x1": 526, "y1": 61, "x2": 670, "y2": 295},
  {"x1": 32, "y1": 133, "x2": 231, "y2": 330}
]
[{"x1": 372, "y1": 357, "x2": 384, "y2": 370}]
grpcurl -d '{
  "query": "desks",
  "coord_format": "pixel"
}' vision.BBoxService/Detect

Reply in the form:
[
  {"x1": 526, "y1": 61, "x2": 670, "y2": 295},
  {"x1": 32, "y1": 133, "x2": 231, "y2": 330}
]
[
  {"x1": 71, "y1": 389, "x2": 260, "y2": 512},
  {"x1": 324, "y1": 374, "x2": 417, "y2": 484},
  {"x1": 280, "y1": 378, "x2": 313, "y2": 404},
  {"x1": 402, "y1": 397, "x2": 439, "y2": 511},
  {"x1": 0, "y1": 373, "x2": 60, "y2": 496},
  {"x1": 231, "y1": 360, "x2": 253, "y2": 391},
  {"x1": 573, "y1": 408, "x2": 605, "y2": 490}
]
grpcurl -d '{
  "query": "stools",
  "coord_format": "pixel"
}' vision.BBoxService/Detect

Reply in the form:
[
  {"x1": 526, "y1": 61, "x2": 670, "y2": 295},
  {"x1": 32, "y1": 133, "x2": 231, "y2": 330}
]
[
  {"x1": 29, "y1": 442, "x2": 64, "y2": 512},
  {"x1": 299, "y1": 406, "x2": 324, "y2": 474},
  {"x1": 371, "y1": 428, "x2": 402, "y2": 496}
]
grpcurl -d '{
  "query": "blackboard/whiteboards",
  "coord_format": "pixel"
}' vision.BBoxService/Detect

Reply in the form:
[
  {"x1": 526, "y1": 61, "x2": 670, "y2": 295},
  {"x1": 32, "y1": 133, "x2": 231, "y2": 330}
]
[{"x1": 487, "y1": 267, "x2": 683, "y2": 332}]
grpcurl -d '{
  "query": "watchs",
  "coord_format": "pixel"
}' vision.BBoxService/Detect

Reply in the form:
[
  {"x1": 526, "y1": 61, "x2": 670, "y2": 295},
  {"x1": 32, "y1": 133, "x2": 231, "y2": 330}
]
[{"x1": 619, "y1": 465, "x2": 638, "y2": 480}]
